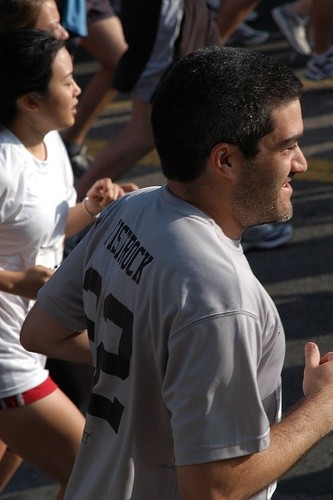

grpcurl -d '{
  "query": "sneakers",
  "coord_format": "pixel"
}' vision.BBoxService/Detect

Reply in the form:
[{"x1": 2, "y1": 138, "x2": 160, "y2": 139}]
[
  {"x1": 69, "y1": 145, "x2": 95, "y2": 180},
  {"x1": 271, "y1": 5, "x2": 312, "y2": 56},
  {"x1": 208, "y1": 1, "x2": 270, "y2": 47},
  {"x1": 241, "y1": 223, "x2": 294, "y2": 250},
  {"x1": 304, "y1": 52, "x2": 333, "y2": 81}
]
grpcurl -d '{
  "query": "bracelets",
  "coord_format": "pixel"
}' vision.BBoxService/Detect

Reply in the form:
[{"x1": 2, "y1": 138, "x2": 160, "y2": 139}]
[{"x1": 82, "y1": 197, "x2": 100, "y2": 217}]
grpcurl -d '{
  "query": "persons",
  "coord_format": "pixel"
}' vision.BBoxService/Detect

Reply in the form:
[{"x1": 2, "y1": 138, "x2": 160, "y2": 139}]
[
  {"x1": 0, "y1": 29, "x2": 137, "y2": 500},
  {"x1": 20, "y1": 47, "x2": 333, "y2": 500},
  {"x1": 0, "y1": 0, "x2": 333, "y2": 250}
]
